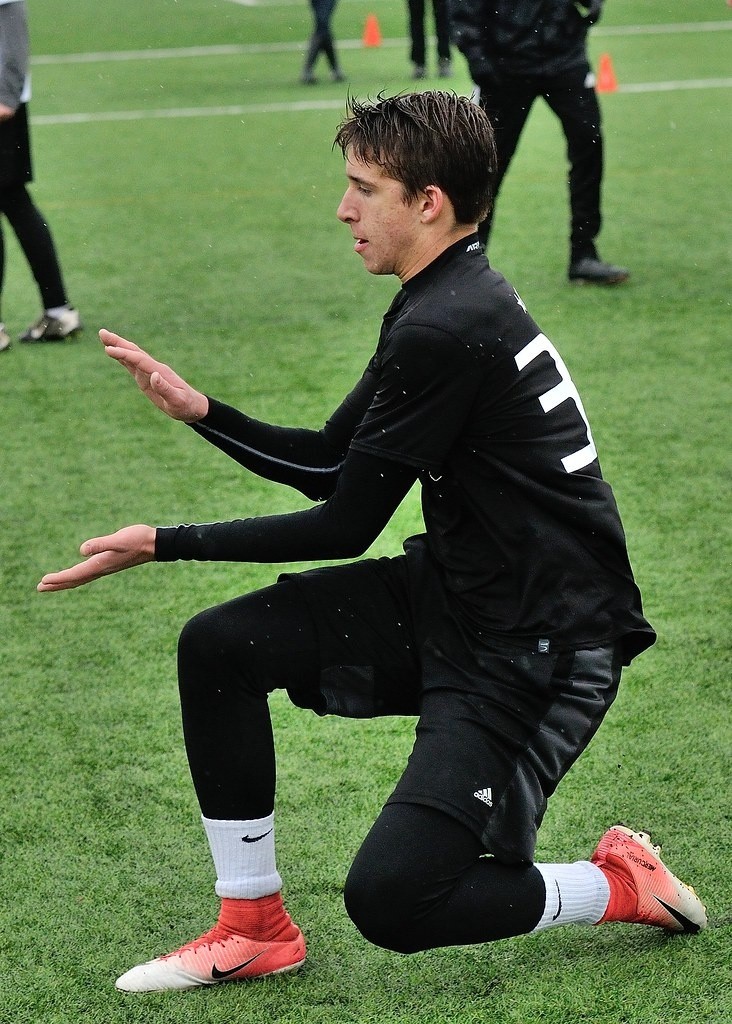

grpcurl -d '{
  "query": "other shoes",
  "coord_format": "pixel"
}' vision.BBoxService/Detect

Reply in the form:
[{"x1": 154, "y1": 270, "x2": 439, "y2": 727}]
[
  {"x1": 412, "y1": 67, "x2": 427, "y2": 79},
  {"x1": 329, "y1": 72, "x2": 348, "y2": 82},
  {"x1": 437, "y1": 68, "x2": 451, "y2": 79},
  {"x1": 300, "y1": 76, "x2": 321, "y2": 86}
]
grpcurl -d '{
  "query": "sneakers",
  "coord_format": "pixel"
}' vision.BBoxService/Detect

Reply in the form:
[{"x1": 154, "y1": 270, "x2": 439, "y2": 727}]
[
  {"x1": 115, "y1": 913, "x2": 307, "y2": 996},
  {"x1": 18, "y1": 307, "x2": 85, "y2": 346},
  {"x1": 589, "y1": 824, "x2": 708, "y2": 934},
  {"x1": 569, "y1": 257, "x2": 632, "y2": 286},
  {"x1": 0, "y1": 322, "x2": 12, "y2": 351}
]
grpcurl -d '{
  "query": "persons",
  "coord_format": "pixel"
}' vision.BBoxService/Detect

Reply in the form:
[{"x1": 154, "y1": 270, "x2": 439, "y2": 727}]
[
  {"x1": 448, "y1": 0, "x2": 629, "y2": 284},
  {"x1": 35, "y1": 91, "x2": 708, "y2": 995},
  {"x1": 0, "y1": 0, "x2": 81, "y2": 351},
  {"x1": 408, "y1": 0, "x2": 452, "y2": 80},
  {"x1": 301, "y1": 0, "x2": 346, "y2": 84}
]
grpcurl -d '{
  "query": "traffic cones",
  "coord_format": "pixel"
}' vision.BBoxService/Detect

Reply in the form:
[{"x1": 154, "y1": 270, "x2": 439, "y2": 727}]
[
  {"x1": 364, "y1": 15, "x2": 379, "y2": 46},
  {"x1": 596, "y1": 54, "x2": 618, "y2": 93}
]
